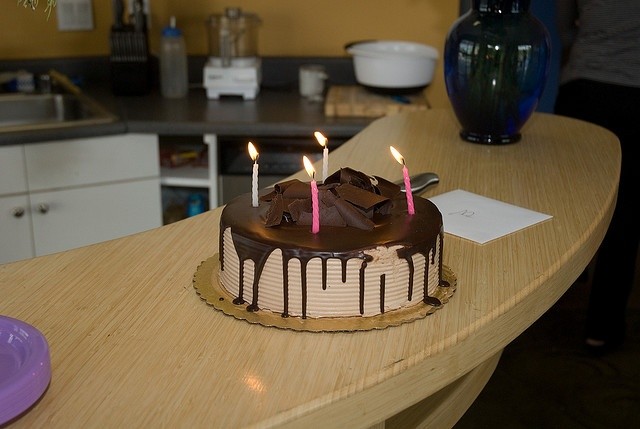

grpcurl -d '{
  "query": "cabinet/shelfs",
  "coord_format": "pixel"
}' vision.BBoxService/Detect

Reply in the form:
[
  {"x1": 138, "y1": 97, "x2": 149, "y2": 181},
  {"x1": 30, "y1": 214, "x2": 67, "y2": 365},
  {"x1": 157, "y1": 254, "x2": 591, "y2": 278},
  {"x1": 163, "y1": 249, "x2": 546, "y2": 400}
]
[{"x1": 0, "y1": 133, "x2": 163, "y2": 260}]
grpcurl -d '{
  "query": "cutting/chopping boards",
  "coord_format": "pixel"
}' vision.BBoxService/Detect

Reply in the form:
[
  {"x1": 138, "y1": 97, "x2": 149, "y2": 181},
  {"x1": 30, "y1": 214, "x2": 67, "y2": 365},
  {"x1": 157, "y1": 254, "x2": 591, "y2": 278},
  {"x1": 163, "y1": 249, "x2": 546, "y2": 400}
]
[{"x1": 324, "y1": 81, "x2": 432, "y2": 115}]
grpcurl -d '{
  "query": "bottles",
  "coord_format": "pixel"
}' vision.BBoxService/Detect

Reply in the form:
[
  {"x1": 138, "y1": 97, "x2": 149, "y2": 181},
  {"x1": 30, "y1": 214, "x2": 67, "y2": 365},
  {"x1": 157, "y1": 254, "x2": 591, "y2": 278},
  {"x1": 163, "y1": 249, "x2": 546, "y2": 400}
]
[
  {"x1": 443, "y1": 0, "x2": 552, "y2": 146},
  {"x1": 159, "y1": 16, "x2": 189, "y2": 100}
]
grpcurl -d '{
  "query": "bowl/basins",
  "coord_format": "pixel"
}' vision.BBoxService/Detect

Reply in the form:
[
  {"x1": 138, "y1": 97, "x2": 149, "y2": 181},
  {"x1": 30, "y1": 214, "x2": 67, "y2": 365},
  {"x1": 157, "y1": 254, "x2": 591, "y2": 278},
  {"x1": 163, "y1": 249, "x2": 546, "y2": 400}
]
[{"x1": 345, "y1": 40, "x2": 439, "y2": 88}]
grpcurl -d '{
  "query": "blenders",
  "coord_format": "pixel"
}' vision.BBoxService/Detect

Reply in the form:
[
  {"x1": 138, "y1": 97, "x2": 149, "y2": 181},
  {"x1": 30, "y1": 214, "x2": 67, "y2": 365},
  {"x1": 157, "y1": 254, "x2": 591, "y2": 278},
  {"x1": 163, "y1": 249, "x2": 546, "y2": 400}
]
[{"x1": 203, "y1": 6, "x2": 263, "y2": 101}]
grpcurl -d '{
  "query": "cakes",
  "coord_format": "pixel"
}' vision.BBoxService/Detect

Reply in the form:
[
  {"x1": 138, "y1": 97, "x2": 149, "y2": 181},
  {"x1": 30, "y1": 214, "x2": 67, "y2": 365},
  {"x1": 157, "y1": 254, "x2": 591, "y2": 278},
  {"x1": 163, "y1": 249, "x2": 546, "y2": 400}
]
[{"x1": 217, "y1": 167, "x2": 444, "y2": 319}]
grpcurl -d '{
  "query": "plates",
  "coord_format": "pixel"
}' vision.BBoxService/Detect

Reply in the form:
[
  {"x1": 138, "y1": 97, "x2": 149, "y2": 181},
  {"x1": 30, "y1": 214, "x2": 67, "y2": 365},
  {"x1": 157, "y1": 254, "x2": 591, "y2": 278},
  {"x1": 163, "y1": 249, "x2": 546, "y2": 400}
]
[{"x1": 0, "y1": 316, "x2": 52, "y2": 426}]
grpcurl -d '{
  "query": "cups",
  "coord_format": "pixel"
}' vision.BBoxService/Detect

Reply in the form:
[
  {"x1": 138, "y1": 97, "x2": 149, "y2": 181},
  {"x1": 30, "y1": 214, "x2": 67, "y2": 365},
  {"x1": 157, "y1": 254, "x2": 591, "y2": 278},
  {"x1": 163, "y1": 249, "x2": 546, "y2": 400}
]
[{"x1": 298, "y1": 64, "x2": 328, "y2": 97}]
